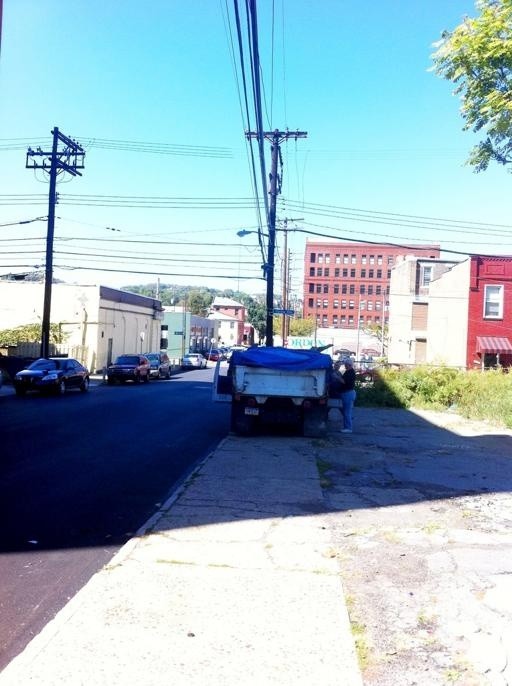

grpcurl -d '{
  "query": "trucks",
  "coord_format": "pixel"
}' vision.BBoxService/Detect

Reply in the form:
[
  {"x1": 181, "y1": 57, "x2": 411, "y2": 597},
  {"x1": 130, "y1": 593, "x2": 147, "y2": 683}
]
[{"x1": 211, "y1": 346, "x2": 333, "y2": 437}]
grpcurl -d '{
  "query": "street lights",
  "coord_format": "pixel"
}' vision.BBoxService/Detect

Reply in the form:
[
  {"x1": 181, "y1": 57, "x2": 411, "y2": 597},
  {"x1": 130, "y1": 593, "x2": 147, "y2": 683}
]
[{"x1": 236, "y1": 230, "x2": 275, "y2": 346}]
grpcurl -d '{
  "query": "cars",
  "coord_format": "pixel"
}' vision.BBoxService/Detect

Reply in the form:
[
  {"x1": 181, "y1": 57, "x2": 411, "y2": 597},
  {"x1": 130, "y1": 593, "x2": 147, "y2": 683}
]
[
  {"x1": 180, "y1": 344, "x2": 248, "y2": 369},
  {"x1": 355, "y1": 362, "x2": 403, "y2": 382},
  {"x1": 334, "y1": 348, "x2": 382, "y2": 356},
  {"x1": 14, "y1": 357, "x2": 91, "y2": 396},
  {"x1": 107, "y1": 353, "x2": 173, "y2": 384}
]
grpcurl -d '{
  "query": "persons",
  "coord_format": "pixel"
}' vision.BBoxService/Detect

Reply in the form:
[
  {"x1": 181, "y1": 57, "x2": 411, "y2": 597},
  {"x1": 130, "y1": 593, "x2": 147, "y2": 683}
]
[{"x1": 330, "y1": 359, "x2": 356, "y2": 433}]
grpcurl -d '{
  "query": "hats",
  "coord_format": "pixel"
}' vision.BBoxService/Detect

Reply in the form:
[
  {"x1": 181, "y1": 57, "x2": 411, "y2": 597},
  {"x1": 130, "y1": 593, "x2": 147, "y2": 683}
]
[{"x1": 342, "y1": 358, "x2": 353, "y2": 365}]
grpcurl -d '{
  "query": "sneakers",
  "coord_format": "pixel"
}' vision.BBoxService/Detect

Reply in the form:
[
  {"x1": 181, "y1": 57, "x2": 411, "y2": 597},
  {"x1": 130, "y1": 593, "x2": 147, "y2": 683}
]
[{"x1": 341, "y1": 429, "x2": 353, "y2": 433}]
